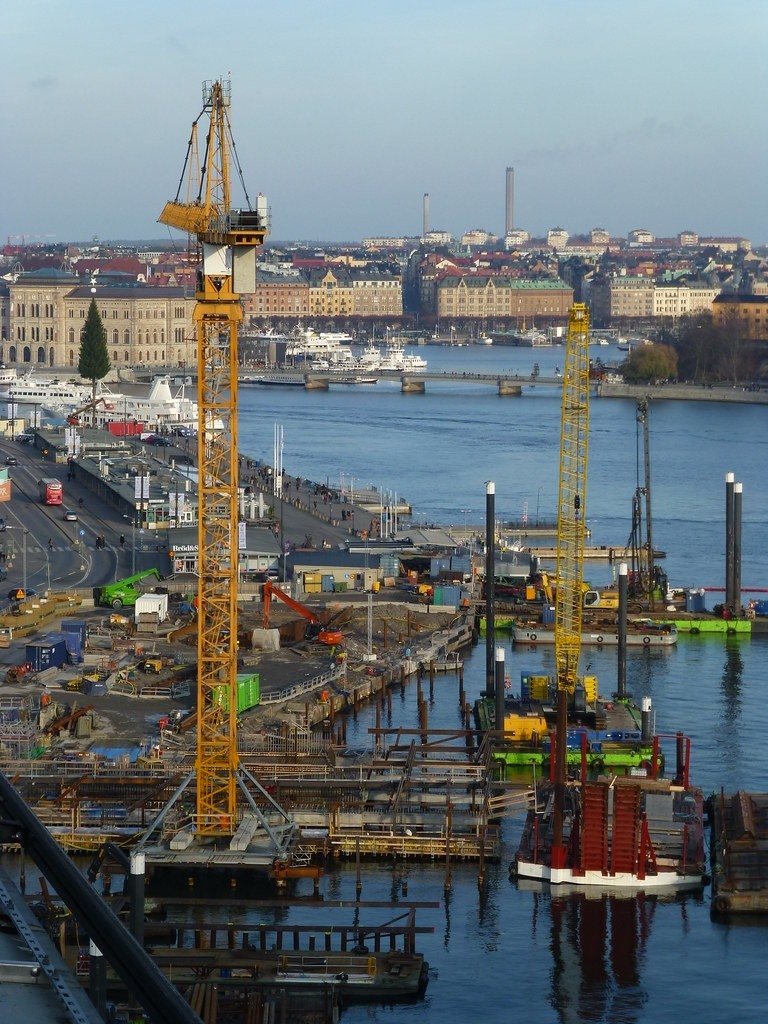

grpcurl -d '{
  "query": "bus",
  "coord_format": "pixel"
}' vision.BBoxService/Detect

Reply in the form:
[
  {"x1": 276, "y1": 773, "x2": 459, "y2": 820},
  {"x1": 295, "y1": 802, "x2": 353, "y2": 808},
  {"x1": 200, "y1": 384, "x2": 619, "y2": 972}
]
[{"x1": 37, "y1": 476, "x2": 65, "y2": 506}]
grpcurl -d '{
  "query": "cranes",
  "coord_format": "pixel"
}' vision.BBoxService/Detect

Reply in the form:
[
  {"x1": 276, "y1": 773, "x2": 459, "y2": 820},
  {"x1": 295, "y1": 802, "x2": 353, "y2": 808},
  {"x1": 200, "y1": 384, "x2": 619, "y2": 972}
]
[
  {"x1": 556, "y1": 304, "x2": 591, "y2": 700},
  {"x1": 161, "y1": 75, "x2": 271, "y2": 832}
]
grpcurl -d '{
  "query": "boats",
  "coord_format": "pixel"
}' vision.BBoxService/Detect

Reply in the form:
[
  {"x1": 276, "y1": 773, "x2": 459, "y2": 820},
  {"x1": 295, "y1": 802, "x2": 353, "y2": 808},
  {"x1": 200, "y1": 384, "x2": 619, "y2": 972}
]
[
  {"x1": 617, "y1": 346, "x2": 629, "y2": 352},
  {"x1": 473, "y1": 334, "x2": 492, "y2": 345},
  {"x1": 511, "y1": 621, "x2": 678, "y2": 646},
  {"x1": 236, "y1": 331, "x2": 427, "y2": 382},
  {"x1": 597, "y1": 338, "x2": 608, "y2": 345},
  {"x1": 1, "y1": 374, "x2": 96, "y2": 405},
  {"x1": 41, "y1": 375, "x2": 225, "y2": 431}
]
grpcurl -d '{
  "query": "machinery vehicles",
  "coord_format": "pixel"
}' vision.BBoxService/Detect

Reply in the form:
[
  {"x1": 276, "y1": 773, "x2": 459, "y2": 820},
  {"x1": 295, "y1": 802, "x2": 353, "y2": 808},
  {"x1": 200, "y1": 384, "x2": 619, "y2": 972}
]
[
  {"x1": 67, "y1": 397, "x2": 111, "y2": 427},
  {"x1": 253, "y1": 579, "x2": 346, "y2": 660},
  {"x1": 99, "y1": 566, "x2": 168, "y2": 611}
]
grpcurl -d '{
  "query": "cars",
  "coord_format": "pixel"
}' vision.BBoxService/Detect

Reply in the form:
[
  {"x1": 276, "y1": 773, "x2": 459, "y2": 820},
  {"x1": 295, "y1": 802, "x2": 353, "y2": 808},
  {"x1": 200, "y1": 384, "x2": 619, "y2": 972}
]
[
  {"x1": 64, "y1": 511, "x2": 78, "y2": 521},
  {"x1": 9, "y1": 587, "x2": 39, "y2": 602},
  {"x1": 7, "y1": 457, "x2": 17, "y2": 466},
  {"x1": 140, "y1": 433, "x2": 172, "y2": 447}
]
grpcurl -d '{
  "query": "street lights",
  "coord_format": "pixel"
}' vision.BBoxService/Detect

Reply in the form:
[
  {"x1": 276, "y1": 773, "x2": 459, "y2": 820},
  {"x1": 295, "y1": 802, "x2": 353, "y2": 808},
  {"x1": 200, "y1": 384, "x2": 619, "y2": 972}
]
[
  {"x1": 329, "y1": 501, "x2": 331, "y2": 521},
  {"x1": 351, "y1": 510, "x2": 354, "y2": 535},
  {"x1": 124, "y1": 515, "x2": 136, "y2": 575},
  {"x1": 10, "y1": 391, "x2": 17, "y2": 441},
  {"x1": 6, "y1": 525, "x2": 27, "y2": 601},
  {"x1": 308, "y1": 490, "x2": 311, "y2": 512}
]
